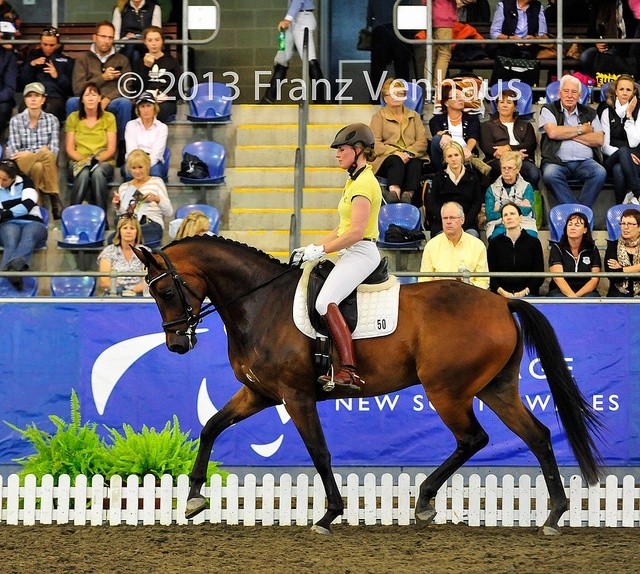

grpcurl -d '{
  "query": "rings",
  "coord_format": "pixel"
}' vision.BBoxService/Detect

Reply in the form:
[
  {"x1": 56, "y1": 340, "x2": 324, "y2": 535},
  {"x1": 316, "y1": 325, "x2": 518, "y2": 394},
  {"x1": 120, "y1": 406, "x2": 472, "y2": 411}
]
[
  {"x1": 148, "y1": 195, "x2": 150, "y2": 197},
  {"x1": 614, "y1": 262, "x2": 616, "y2": 264}
]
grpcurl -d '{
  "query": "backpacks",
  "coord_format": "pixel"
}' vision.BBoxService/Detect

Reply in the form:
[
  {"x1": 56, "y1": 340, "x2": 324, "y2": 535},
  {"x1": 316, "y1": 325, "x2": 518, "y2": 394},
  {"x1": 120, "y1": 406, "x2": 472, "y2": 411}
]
[{"x1": 384, "y1": 223, "x2": 426, "y2": 244}]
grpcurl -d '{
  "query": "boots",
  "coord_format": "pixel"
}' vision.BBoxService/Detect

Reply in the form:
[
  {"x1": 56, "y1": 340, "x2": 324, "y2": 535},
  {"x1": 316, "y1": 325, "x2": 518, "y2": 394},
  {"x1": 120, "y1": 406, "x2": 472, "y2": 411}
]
[
  {"x1": 260, "y1": 64, "x2": 288, "y2": 105},
  {"x1": 309, "y1": 59, "x2": 324, "y2": 104},
  {"x1": 50, "y1": 195, "x2": 63, "y2": 220},
  {"x1": 318, "y1": 302, "x2": 365, "y2": 394}
]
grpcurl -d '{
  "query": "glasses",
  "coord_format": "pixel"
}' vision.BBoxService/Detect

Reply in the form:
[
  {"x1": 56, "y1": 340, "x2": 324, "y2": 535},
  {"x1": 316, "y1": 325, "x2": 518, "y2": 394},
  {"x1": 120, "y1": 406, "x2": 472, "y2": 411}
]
[
  {"x1": 96, "y1": 35, "x2": 114, "y2": 40},
  {"x1": 619, "y1": 222, "x2": 638, "y2": 228},
  {"x1": 41, "y1": 27, "x2": 58, "y2": 36},
  {"x1": 115, "y1": 213, "x2": 138, "y2": 224},
  {"x1": 129, "y1": 194, "x2": 146, "y2": 213},
  {"x1": 442, "y1": 216, "x2": 461, "y2": 220},
  {"x1": 1, "y1": 160, "x2": 16, "y2": 169},
  {"x1": 448, "y1": 94, "x2": 464, "y2": 100},
  {"x1": 501, "y1": 165, "x2": 519, "y2": 173},
  {"x1": 384, "y1": 92, "x2": 406, "y2": 97}
]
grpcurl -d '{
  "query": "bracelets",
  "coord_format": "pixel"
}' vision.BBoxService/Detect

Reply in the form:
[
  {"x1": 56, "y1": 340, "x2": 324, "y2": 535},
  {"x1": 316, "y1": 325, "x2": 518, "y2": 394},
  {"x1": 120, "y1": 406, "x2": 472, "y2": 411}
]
[
  {"x1": 522, "y1": 289, "x2": 528, "y2": 295},
  {"x1": 508, "y1": 35, "x2": 509, "y2": 38},
  {"x1": 626, "y1": 116, "x2": 634, "y2": 121}
]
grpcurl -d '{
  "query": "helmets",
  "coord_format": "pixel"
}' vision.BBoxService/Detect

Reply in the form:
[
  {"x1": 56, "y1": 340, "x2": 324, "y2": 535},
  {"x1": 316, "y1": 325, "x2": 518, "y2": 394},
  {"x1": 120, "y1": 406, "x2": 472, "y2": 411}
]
[{"x1": 330, "y1": 123, "x2": 375, "y2": 149}]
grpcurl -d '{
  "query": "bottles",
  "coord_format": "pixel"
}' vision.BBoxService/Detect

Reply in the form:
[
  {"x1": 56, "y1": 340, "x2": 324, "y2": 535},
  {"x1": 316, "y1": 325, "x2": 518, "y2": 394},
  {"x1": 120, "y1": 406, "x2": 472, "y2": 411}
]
[{"x1": 277, "y1": 27, "x2": 286, "y2": 51}]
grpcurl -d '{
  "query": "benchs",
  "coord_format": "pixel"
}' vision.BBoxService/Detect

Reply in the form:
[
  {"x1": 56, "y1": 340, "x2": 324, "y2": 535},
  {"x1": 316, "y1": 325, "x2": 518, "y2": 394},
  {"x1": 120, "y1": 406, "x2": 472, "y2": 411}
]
[
  {"x1": 450, "y1": 23, "x2": 591, "y2": 69},
  {"x1": 17, "y1": 22, "x2": 178, "y2": 65}
]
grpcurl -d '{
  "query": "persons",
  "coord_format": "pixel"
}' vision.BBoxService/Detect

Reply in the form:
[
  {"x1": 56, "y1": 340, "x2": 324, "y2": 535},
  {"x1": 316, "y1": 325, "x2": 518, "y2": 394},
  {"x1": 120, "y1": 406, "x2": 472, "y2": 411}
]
[
  {"x1": 111, "y1": 148, "x2": 173, "y2": 248},
  {"x1": 429, "y1": 84, "x2": 480, "y2": 157},
  {"x1": 18, "y1": 26, "x2": 74, "y2": 130},
  {"x1": 488, "y1": 0, "x2": 548, "y2": 99},
  {"x1": 546, "y1": 211, "x2": 602, "y2": 297},
  {"x1": 65, "y1": 20, "x2": 132, "y2": 168},
  {"x1": 418, "y1": 200, "x2": 491, "y2": 290},
  {"x1": 485, "y1": 150, "x2": 538, "y2": 242},
  {"x1": 368, "y1": 78, "x2": 428, "y2": 203},
  {"x1": 538, "y1": 73, "x2": 607, "y2": 209},
  {"x1": 0, "y1": 159, "x2": 47, "y2": 290},
  {"x1": 0, "y1": 46, "x2": 18, "y2": 143},
  {"x1": 604, "y1": 208, "x2": 640, "y2": 297},
  {"x1": 132, "y1": 25, "x2": 185, "y2": 122},
  {"x1": 95, "y1": 213, "x2": 152, "y2": 296},
  {"x1": 258, "y1": 0, "x2": 326, "y2": 103},
  {"x1": 600, "y1": 74, "x2": 640, "y2": 204},
  {"x1": 173, "y1": 209, "x2": 209, "y2": 239},
  {"x1": 478, "y1": 89, "x2": 540, "y2": 190},
  {"x1": 418, "y1": 0, "x2": 475, "y2": 104},
  {"x1": 0, "y1": 0, "x2": 24, "y2": 67},
  {"x1": 291, "y1": 123, "x2": 383, "y2": 393},
  {"x1": 595, "y1": 80, "x2": 617, "y2": 123},
  {"x1": 64, "y1": 81, "x2": 118, "y2": 230},
  {"x1": 366, "y1": 0, "x2": 413, "y2": 104},
  {"x1": 487, "y1": 201, "x2": 544, "y2": 298},
  {"x1": 579, "y1": 0, "x2": 636, "y2": 87},
  {"x1": 121, "y1": 91, "x2": 168, "y2": 179},
  {"x1": 427, "y1": 141, "x2": 482, "y2": 238},
  {"x1": 8, "y1": 81, "x2": 63, "y2": 219},
  {"x1": 112, "y1": 0, "x2": 162, "y2": 73}
]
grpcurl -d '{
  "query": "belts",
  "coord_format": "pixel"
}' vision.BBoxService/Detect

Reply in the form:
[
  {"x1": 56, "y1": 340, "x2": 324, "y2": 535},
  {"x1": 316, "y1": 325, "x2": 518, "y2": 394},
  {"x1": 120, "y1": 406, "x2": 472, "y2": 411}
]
[{"x1": 299, "y1": 8, "x2": 316, "y2": 12}]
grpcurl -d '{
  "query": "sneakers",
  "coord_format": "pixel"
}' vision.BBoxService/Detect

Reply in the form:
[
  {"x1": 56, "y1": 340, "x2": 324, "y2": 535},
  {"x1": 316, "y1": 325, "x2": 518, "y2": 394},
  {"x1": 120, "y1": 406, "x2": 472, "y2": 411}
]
[
  {"x1": 401, "y1": 192, "x2": 412, "y2": 204},
  {"x1": 466, "y1": 152, "x2": 492, "y2": 176},
  {"x1": 7, "y1": 276, "x2": 22, "y2": 290},
  {"x1": 386, "y1": 191, "x2": 399, "y2": 204},
  {"x1": 6, "y1": 257, "x2": 25, "y2": 271}
]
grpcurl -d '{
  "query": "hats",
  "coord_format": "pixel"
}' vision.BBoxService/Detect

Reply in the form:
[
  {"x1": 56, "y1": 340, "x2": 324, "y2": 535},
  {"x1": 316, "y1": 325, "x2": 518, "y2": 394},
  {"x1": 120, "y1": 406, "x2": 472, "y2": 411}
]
[
  {"x1": 135, "y1": 92, "x2": 156, "y2": 106},
  {"x1": 22, "y1": 82, "x2": 45, "y2": 97}
]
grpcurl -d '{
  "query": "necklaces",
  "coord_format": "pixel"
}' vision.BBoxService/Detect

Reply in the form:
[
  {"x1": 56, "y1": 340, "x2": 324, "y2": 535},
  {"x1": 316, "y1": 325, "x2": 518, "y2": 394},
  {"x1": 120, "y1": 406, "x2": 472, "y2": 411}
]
[{"x1": 449, "y1": 111, "x2": 462, "y2": 121}]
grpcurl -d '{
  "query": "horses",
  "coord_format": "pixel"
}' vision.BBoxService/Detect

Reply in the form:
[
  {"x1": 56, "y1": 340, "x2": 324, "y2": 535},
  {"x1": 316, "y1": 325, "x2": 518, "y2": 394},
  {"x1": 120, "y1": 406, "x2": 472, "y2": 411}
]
[{"x1": 128, "y1": 234, "x2": 611, "y2": 538}]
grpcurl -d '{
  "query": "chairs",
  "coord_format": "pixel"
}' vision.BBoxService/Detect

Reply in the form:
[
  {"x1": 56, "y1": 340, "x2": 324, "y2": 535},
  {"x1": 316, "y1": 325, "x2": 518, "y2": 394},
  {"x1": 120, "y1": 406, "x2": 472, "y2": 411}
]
[
  {"x1": 376, "y1": 202, "x2": 422, "y2": 253},
  {"x1": 490, "y1": 82, "x2": 535, "y2": 121},
  {"x1": 397, "y1": 270, "x2": 417, "y2": 285},
  {"x1": 1, "y1": 206, "x2": 49, "y2": 252},
  {"x1": 381, "y1": 81, "x2": 426, "y2": 116},
  {"x1": 158, "y1": 149, "x2": 171, "y2": 178},
  {"x1": 184, "y1": 81, "x2": 232, "y2": 127},
  {"x1": 178, "y1": 139, "x2": 227, "y2": 186},
  {"x1": 600, "y1": 81, "x2": 612, "y2": 105},
  {"x1": 605, "y1": 204, "x2": 640, "y2": 246},
  {"x1": 57, "y1": 204, "x2": 106, "y2": 252},
  {"x1": 548, "y1": 204, "x2": 593, "y2": 252},
  {"x1": 0, "y1": 273, "x2": 40, "y2": 298},
  {"x1": 50, "y1": 272, "x2": 97, "y2": 297},
  {"x1": 176, "y1": 204, "x2": 222, "y2": 236},
  {"x1": 545, "y1": 80, "x2": 588, "y2": 107}
]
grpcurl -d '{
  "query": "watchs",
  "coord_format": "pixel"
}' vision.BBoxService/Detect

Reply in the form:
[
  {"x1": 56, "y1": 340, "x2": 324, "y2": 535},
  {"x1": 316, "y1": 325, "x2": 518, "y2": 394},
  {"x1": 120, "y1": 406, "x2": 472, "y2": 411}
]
[{"x1": 577, "y1": 125, "x2": 582, "y2": 135}]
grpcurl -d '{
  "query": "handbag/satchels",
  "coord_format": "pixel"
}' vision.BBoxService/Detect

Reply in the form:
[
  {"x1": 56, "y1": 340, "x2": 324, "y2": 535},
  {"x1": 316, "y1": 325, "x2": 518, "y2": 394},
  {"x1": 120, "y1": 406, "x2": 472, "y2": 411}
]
[
  {"x1": 357, "y1": 26, "x2": 371, "y2": 51},
  {"x1": 525, "y1": 33, "x2": 566, "y2": 60}
]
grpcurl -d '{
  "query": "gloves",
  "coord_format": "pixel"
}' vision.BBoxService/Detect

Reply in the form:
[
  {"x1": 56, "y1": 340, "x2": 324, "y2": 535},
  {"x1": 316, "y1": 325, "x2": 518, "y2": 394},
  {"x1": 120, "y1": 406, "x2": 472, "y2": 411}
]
[
  {"x1": 303, "y1": 245, "x2": 327, "y2": 263},
  {"x1": 290, "y1": 247, "x2": 306, "y2": 265}
]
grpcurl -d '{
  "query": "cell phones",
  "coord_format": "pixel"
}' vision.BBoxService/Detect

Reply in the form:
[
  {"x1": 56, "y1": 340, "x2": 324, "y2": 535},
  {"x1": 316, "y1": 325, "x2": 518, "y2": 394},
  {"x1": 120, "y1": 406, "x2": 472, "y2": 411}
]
[{"x1": 115, "y1": 66, "x2": 123, "y2": 71}]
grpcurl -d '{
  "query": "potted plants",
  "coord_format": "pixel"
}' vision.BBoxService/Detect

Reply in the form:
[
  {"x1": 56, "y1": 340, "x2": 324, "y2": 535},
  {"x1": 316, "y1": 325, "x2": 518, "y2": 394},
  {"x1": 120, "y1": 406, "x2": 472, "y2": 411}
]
[{"x1": 86, "y1": 414, "x2": 230, "y2": 510}]
